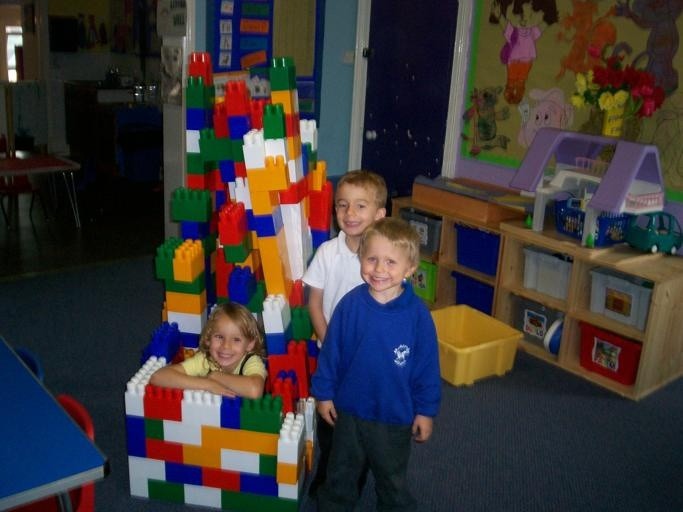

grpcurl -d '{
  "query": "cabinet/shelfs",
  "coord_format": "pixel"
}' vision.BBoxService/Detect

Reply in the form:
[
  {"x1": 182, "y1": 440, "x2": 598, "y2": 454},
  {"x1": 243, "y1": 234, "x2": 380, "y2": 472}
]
[
  {"x1": 391, "y1": 195, "x2": 527, "y2": 321},
  {"x1": 493, "y1": 216, "x2": 682, "y2": 404}
]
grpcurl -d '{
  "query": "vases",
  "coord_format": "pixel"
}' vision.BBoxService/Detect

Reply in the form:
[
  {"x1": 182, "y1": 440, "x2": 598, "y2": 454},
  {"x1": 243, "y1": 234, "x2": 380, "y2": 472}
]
[{"x1": 601, "y1": 105, "x2": 624, "y2": 138}]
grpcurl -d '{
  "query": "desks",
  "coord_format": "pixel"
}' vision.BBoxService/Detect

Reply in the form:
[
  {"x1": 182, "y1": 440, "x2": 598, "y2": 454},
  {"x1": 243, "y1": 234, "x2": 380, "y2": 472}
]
[
  {"x1": 0, "y1": 154, "x2": 84, "y2": 233},
  {"x1": 1, "y1": 333, "x2": 112, "y2": 511}
]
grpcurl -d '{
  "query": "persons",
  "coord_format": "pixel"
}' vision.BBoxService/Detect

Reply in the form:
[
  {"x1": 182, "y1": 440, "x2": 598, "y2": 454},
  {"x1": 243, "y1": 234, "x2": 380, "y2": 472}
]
[
  {"x1": 148, "y1": 302, "x2": 267, "y2": 399},
  {"x1": 309, "y1": 214, "x2": 441, "y2": 511},
  {"x1": 300, "y1": 169, "x2": 390, "y2": 502}
]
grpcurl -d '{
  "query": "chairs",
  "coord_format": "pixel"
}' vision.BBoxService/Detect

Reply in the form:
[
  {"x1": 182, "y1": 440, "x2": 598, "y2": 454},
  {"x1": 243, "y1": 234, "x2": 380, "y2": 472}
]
[
  {"x1": 6, "y1": 346, "x2": 97, "y2": 511},
  {"x1": 0, "y1": 132, "x2": 39, "y2": 232}
]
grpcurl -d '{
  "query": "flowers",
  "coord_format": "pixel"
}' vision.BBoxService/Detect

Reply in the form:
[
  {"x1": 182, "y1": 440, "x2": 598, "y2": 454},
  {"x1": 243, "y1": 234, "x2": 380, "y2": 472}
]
[{"x1": 571, "y1": 57, "x2": 666, "y2": 121}]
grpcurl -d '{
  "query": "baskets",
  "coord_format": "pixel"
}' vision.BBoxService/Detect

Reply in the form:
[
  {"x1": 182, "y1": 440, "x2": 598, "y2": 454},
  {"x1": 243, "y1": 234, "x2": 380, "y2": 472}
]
[{"x1": 553, "y1": 197, "x2": 632, "y2": 248}]
